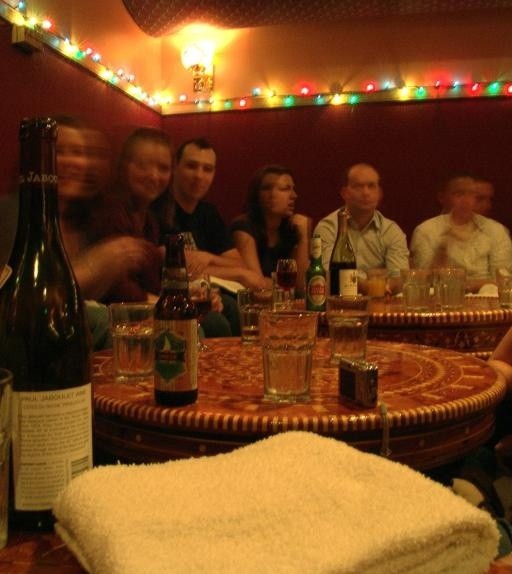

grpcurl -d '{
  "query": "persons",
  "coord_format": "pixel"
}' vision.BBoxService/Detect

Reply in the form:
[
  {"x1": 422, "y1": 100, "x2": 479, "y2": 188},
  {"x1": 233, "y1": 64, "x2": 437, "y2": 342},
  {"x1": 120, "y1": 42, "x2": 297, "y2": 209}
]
[
  {"x1": 409, "y1": 162, "x2": 511, "y2": 278},
  {"x1": 1, "y1": 113, "x2": 153, "y2": 302},
  {"x1": 475, "y1": 174, "x2": 494, "y2": 217},
  {"x1": 149, "y1": 136, "x2": 265, "y2": 294},
  {"x1": 307, "y1": 159, "x2": 410, "y2": 292},
  {"x1": 85, "y1": 124, "x2": 176, "y2": 305},
  {"x1": 231, "y1": 164, "x2": 312, "y2": 301}
]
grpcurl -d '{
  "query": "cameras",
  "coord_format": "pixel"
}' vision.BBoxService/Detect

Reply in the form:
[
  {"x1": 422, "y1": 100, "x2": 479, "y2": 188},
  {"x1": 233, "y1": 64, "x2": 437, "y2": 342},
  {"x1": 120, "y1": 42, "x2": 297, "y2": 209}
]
[{"x1": 337, "y1": 354, "x2": 380, "y2": 407}]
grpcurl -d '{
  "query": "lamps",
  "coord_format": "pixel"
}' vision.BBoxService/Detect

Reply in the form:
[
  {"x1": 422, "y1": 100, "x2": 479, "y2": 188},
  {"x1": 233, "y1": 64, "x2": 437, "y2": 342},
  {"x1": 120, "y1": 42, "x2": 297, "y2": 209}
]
[{"x1": 176, "y1": 24, "x2": 220, "y2": 91}]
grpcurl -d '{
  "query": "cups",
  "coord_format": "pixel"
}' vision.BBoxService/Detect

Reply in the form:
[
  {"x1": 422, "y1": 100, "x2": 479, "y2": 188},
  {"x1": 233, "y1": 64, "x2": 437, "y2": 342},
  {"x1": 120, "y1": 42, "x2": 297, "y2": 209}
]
[
  {"x1": 198, "y1": 273, "x2": 212, "y2": 315},
  {"x1": 108, "y1": 302, "x2": 156, "y2": 374},
  {"x1": 0, "y1": 370, "x2": 14, "y2": 548},
  {"x1": 325, "y1": 293, "x2": 370, "y2": 363},
  {"x1": 400, "y1": 269, "x2": 512, "y2": 312},
  {"x1": 236, "y1": 271, "x2": 294, "y2": 343},
  {"x1": 259, "y1": 310, "x2": 320, "y2": 403}
]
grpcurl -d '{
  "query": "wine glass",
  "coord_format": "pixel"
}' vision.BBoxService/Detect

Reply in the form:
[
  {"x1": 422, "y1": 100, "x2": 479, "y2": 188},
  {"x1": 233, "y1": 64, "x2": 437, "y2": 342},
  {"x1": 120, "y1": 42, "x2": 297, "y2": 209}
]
[
  {"x1": 277, "y1": 260, "x2": 298, "y2": 311},
  {"x1": 385, "y1": 268, "x2": 404, "y2": 313},
  {"x1": 178, "y1": 231, "x2": 199, "y2": 300}
]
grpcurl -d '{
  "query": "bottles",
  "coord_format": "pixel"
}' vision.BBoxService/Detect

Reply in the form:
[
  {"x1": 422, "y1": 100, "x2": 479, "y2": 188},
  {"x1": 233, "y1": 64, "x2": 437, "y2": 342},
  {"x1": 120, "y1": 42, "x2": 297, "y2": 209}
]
[
  {"x1": 155, "y1": 233, "x2": 199, "y2": 407},
  {"x1": 304, "y1": 208, "x2": 357, "y2": 311},
  {"x1": 0, "y1": 117, "x2": 97, "y2": 541}
]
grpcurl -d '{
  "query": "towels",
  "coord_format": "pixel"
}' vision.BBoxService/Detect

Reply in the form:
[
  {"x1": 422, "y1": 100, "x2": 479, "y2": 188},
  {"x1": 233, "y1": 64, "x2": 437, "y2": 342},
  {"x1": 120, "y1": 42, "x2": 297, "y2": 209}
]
[{"x1": 50, "y1": 429, "x2": 500, "y2": 574}]
